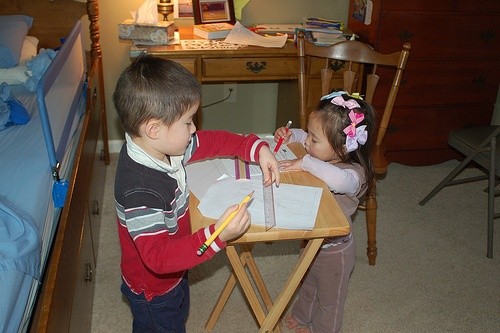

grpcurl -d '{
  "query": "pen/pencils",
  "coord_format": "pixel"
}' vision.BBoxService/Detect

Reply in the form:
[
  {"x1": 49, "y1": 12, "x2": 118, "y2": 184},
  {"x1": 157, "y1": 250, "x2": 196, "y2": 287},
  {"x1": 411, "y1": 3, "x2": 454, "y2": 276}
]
[
  {"x1": 196, "y1": 190, "x2": 254, "y2": 256},
  {"x1": 264, "y1": 32, "x2": 295, "y2": 42},
  {"x1": 273, "y1": 120, "x2": 292, "y2": 156}
]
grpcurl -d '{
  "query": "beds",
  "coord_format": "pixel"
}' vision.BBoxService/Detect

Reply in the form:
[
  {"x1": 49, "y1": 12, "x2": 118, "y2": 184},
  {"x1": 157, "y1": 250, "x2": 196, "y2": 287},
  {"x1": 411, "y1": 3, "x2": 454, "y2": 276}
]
[{"x1": 0, "y1": 0, "x2": 114, "y2": 333}]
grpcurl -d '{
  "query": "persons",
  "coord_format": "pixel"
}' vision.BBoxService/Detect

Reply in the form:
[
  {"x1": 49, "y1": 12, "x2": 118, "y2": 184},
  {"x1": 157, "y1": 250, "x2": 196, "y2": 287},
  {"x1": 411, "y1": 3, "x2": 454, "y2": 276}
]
[
  {"x1": 271, "y1": 89, "x2": 372, "y2": 333},
  {"x1": 112, "y1": 56, "x2": 280, "y2": 332}
]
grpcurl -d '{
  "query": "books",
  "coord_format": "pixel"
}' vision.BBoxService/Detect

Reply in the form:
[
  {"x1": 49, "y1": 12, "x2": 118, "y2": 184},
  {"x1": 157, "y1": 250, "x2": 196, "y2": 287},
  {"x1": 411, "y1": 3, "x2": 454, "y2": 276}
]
[
  {"x1": 295, "y1": 14, "x2": 346, "y2": 47},
  {"x1": 192, "y1": 22, "x2": 234, "y2": 41}
]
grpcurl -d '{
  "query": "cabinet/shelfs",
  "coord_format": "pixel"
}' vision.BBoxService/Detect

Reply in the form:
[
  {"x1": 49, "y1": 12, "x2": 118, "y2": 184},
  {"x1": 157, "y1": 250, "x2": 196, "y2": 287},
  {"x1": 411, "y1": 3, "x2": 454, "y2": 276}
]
[
  {"x1": 129, "y1": 33, "x2": 364, "y2": 133},
  {"x1": 348, "y1": 0, "x2": 500, "y2": 167}
]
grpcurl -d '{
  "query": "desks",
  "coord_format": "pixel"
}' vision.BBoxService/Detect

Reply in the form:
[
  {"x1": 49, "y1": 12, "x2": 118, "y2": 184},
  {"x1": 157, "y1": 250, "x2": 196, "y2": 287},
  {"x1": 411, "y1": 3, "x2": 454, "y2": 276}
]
[{"x1": 188, "y1": 141, "x2": 351, "y2": 333}]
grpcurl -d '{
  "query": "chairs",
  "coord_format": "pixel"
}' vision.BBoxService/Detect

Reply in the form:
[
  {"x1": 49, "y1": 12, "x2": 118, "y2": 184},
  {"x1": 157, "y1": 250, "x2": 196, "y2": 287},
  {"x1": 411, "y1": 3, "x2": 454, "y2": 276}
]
[
  {"x1": 264, "y1": 31, "x2": 411, "y2": 265},
  {"x1": 418, "y1": 126, "x2": 500, "y2": 258}
]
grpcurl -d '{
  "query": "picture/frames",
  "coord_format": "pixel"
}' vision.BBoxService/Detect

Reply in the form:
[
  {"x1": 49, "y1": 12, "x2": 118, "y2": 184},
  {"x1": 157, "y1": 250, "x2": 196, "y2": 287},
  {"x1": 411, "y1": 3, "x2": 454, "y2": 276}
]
[{"x1": 192, "y1": 0, "x2": 236, "y2": 25}]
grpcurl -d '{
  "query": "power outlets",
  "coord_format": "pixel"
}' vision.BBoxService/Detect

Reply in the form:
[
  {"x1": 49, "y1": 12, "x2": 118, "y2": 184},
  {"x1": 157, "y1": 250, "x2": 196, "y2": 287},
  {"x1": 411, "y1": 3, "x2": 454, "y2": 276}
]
[{"x1": 223, "y1": 82, "x2": 237, "y2": 103}]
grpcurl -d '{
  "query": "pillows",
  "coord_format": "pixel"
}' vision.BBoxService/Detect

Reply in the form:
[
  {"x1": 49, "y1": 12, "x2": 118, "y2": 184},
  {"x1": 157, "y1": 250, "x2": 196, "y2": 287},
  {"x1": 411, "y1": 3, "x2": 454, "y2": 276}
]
[{"x1": 0, "y1": 15, "x2": 34, "y2": 68}]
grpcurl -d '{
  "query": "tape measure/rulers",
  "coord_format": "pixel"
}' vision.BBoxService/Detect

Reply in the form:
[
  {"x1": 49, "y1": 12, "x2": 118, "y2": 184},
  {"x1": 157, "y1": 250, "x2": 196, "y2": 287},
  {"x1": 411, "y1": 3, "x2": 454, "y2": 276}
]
[{"x1": 262, "y1": 167, "x2": 276, "y2": 232}]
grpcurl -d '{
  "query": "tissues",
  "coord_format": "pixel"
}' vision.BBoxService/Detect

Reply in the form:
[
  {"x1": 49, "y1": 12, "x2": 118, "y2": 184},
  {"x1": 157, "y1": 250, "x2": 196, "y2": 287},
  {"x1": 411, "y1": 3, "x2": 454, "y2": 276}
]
[{"x1": 117, "y1": 2, "x2": 175, "y2": 43}]
